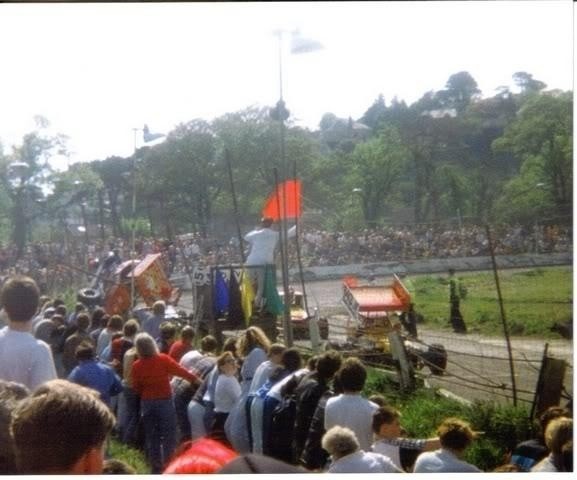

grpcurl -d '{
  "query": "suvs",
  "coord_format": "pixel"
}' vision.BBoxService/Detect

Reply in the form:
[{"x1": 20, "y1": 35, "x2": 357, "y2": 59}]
[{"x1": 275, "y1": 290, "x2": 328, "y2": 338}]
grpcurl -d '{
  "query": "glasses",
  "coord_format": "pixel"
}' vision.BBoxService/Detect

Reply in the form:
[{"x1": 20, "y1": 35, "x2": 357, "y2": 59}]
[{"x1": 224, "y1": 358, "x2": 239, "y2": 365}]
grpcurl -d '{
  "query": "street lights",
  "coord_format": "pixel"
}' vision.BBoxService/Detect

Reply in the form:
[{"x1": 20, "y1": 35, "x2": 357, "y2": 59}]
[
  {"x1": 267, "y1": 96, "x2": 296, "y2": 351},
  {"x1": 350, "y1": 188, "x2": 371, "y2": 234},
  {"x1": 130, "y1": 124, "x2": 168, "y2": 310},
  {"x1": 509, "y1": 182, "x2": 546, "y2": 198}
]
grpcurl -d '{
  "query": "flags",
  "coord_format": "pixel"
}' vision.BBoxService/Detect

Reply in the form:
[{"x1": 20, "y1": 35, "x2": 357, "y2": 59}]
[{"x1": 262, "y1": 181, "x2": 301, "y2": 221}]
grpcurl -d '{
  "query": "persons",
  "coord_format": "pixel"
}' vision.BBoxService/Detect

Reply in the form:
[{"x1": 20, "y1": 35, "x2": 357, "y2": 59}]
[
  {"x1": 246, "y1": 218, "x2": 281, "y2": 315},
  {"x1": 261, "y1": 351, "x2": 483, "y2": 473},
  {"x1": 1, "y1": 238, "x2": 252, "y2": 284},
  {"x1": 1, "y1": 275, "x2": 300, "y2": 474},
  {"x1": 447, "y1": 268, "x2": 468, "y2": 332},
  {"x1": 274, "y1": 220, "x2": 574, "y2": 267},
  {"x1": 492, "y1": 405, "x2": 573, "y2": 473}
]
